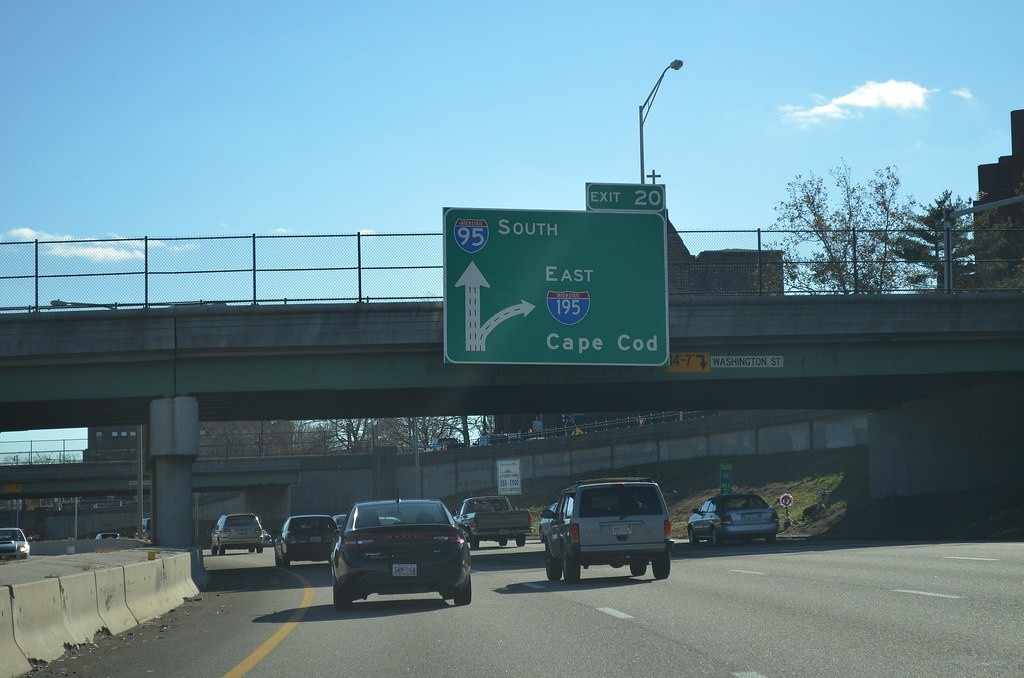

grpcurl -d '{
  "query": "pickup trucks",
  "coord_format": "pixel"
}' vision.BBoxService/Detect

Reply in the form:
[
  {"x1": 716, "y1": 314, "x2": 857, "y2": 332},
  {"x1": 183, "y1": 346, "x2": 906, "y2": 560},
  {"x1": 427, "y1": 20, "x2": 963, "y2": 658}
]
[
  {"x1": 453, "y1": 494, "x2": 531, "y2": 550},
  {"x1": 423, "y1": 437, "x2": 470, "y2": 452}
]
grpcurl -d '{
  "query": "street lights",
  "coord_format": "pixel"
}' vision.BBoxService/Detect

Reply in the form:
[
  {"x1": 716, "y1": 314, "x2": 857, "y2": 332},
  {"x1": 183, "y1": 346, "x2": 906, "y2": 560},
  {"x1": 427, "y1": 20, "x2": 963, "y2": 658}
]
[{"x1": 639, "y1": 59, "x2": 685, "y2": 184}]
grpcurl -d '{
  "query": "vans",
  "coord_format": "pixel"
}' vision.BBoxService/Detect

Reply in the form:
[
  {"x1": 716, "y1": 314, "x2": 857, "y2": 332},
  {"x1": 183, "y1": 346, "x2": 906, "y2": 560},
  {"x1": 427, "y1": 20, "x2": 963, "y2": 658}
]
[{"x1": 137, "y1": 519, "x2": 151, "y2": 540}]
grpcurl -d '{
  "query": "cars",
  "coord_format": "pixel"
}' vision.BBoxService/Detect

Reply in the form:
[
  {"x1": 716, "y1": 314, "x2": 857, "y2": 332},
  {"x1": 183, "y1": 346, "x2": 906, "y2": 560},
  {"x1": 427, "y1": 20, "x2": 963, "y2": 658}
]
[
  {"x1": 95, "y1": 533, "x2": 120, "y2": 540},
  {"x1": 275, "y1": 515, "x2": 341, "y2": 567},
  {"x1": 332, "y1": 514, "x2": 348, "y2": 532},
  {"x1": 329, "y1": 499, "x2": 472, "y2": 612},
  {"x1": 262, "y1": 530, "x2": 272, "y2": 547},
  {"x1": 539, "y1": 503, "x2": 557, "y2": 542},
  {"x1": 0, "y1": 528, "x2": 33, "y2": 559},
  {"x1": 687, "y1": 491, "x2": 780, "y2": 548},
  {"x1": 475, "y1": 433, "x2": 511, "y2": 446},
  {"x1": 380, "y1": 516, "x2": 402, "y2": 526}
]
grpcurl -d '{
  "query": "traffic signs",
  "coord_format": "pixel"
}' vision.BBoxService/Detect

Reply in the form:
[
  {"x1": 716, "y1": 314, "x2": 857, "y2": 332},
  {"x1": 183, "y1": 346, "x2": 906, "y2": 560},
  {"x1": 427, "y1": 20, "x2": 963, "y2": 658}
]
[
  {"x1": 665, "y1": 352, "x2": 711, "y2": 373},
  {"x1": 438, "y1": 206, "x2": 673, "y2": 371}
]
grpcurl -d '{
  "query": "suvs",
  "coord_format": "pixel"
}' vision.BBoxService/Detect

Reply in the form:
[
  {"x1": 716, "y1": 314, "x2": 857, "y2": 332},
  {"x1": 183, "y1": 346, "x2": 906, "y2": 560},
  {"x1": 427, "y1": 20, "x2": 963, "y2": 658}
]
[
  {"x1": 539, "y1": 477, "x2": 675, "y2": 584},
  {"x1": 210, "y1": 513, "x2": 263, "y2": 556}
]
